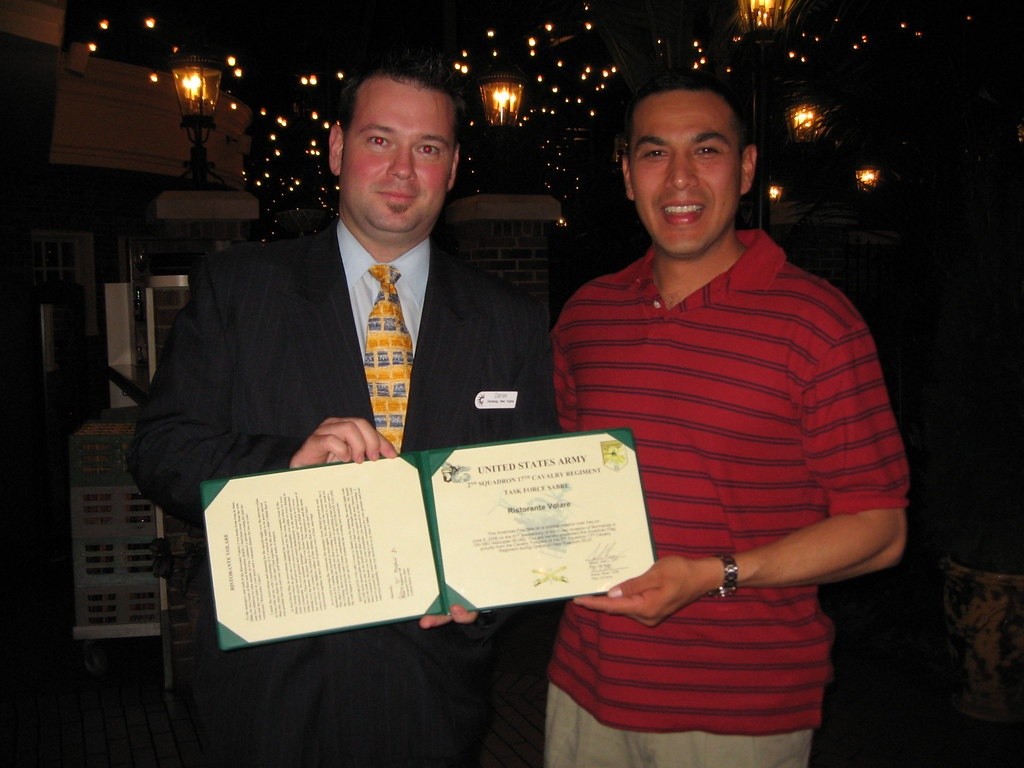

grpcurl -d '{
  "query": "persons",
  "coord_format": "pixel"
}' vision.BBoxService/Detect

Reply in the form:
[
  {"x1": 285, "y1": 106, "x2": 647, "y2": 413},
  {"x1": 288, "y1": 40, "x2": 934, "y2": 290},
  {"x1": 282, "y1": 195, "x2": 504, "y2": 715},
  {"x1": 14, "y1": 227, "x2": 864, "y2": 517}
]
[
  {"x1": 539, "y1": 73, "x2": 909, "y2": 768},
  {"x1": 121, "y1": 42, "x2": 559, "y2": 768}
]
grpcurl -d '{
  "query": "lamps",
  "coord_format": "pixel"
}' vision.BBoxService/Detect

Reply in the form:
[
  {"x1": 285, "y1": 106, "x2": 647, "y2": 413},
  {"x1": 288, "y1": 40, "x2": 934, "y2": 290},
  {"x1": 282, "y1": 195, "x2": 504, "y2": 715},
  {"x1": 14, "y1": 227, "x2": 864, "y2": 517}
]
[
  {"x1": 473, "y1": 50, "x2": 530, "y2": 194},
  {"x1": 737, "y1": 0, "x2": 803, "y2": 47},
  {"x1": 168, "y1": 29, "x2": 232, "y2": 192}
]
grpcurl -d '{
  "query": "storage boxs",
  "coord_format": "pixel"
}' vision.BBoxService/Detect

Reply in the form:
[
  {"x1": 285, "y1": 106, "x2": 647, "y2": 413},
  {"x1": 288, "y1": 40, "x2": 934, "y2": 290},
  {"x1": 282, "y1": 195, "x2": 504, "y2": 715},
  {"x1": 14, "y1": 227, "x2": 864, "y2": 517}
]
[{"x1": 69, "y1": 420, "x2": 161, "y2": 627}]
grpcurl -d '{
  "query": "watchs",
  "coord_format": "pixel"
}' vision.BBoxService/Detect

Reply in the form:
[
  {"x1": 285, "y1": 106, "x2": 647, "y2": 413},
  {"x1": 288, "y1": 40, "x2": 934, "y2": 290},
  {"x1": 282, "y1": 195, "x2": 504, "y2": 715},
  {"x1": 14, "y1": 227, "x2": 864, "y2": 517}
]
[{"x1": 708, "y1": 555, "x2": 738, "y2": 597}]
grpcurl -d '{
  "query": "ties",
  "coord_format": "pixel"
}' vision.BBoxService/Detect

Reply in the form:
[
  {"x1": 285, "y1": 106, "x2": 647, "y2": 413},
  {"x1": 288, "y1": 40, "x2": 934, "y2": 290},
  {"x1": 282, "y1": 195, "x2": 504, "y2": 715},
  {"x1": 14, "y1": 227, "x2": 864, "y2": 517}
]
[{"x1": 364, "y1": 264, "x2": 414, "y2": 454}]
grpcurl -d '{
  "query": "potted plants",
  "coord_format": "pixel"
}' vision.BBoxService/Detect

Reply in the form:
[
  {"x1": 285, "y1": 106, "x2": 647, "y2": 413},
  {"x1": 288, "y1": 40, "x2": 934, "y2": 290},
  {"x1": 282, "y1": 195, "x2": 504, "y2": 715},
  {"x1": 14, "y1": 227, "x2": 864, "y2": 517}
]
[{"x1": 916, "y1": 141, "x2": 1024, "y2": 726}]
